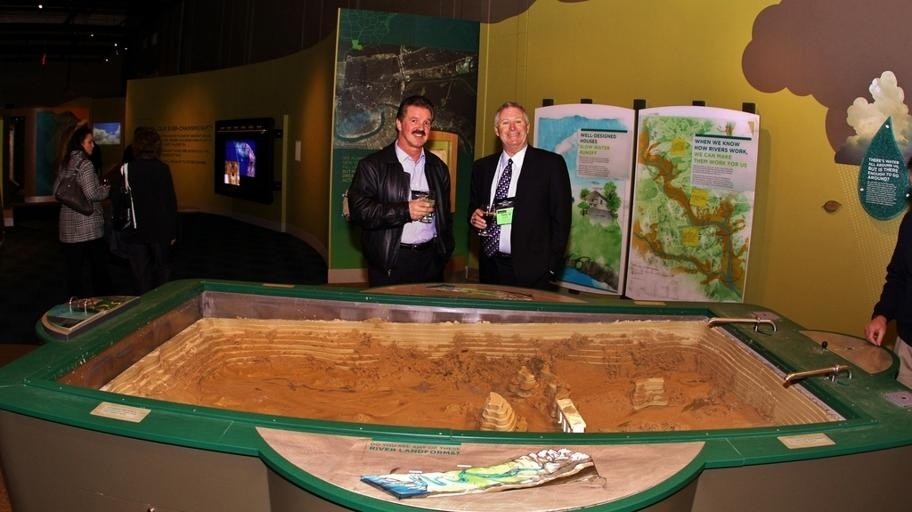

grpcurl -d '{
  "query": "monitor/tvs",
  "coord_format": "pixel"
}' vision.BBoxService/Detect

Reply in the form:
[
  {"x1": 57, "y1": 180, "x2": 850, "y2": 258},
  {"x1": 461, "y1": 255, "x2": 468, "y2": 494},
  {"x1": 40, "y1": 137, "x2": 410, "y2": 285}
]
[{"x1": 215, "y1": 117, "x2": 274, "y2": 204}]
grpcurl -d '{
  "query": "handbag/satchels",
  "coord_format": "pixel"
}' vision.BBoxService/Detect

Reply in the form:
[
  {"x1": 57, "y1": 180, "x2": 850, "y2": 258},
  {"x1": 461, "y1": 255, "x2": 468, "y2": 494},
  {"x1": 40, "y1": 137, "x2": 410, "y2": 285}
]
[{"x1": 55, "y1": 177, "x2": 94, "y2": 217}]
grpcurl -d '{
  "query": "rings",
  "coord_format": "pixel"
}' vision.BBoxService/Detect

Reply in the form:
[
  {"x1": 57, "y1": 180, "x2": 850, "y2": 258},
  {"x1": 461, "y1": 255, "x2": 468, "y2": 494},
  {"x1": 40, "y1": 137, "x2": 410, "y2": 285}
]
[
  {"x1": 472, "y1": 219, "x2": 476, "y2": 224},
  {"x1": 473, "y1": 215, "x2": 477, "y2": 220}
]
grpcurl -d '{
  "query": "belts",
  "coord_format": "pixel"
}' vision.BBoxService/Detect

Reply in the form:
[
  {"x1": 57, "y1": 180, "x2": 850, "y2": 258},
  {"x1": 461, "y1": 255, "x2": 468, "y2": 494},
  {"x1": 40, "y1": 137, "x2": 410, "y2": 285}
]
[{"x1": 399, "y1": 238, "x2": 434, "y2": 250}]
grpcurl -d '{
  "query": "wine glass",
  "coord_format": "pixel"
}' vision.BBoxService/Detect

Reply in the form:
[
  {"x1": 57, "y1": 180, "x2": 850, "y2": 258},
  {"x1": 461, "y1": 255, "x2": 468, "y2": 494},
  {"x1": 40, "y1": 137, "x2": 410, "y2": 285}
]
[
  {"x1": 477, "y1": 202, "x2": 495, "y2": 237},
  {"x1": 418, "y1": 190, "x2": 438, "y2": 224}
]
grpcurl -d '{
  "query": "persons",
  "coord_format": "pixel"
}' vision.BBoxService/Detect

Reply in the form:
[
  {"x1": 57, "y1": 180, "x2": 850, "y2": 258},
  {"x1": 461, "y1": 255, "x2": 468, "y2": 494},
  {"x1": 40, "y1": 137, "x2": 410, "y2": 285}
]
[
  {"x1": 224, "y1": 141, "x2": 257, "y2": 186},
  {"x1": 348, "y1": 94, "x2": 456, "y2": 289},
  {"x1": 466, "y1": 101, "x2": 573, "y2": 296},
  {"x1": 53, "y1": 126, "x2": 116, "y2": 297},
  {"x1": 865, "y1": 156, "x2": 911, "y2": 391},
  {"x1": 109, "y1": 128, "x2": 178, "y2": 292}
]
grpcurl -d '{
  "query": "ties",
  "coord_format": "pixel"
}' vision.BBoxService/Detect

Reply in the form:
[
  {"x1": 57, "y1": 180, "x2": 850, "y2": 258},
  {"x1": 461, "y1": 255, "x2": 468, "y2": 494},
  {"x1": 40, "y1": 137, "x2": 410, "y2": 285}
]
[{"x1": 484, "y1": 158, "x2": 514, "y2": 258}]
